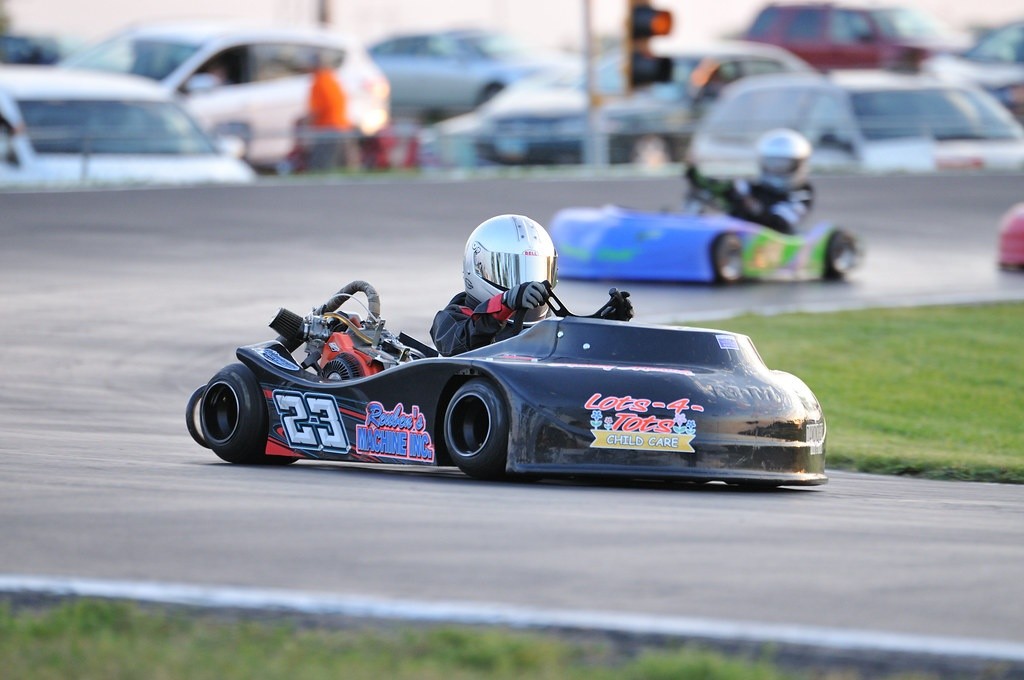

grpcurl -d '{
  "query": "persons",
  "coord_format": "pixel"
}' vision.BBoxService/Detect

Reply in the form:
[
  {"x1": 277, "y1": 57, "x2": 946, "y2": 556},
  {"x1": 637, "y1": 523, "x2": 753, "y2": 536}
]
[
  {"x1": 430, "y1": 214, "x2": 633, "y2": 358},
  {"x1": 276, "y1": 51, "x2": 348, "y2": 173},
  {"x1": 685, "y1": 127, "x2": 814, "y2": 234},
  {"x1": 203, "y1": 54, "x2": 233, "y2": 88}
]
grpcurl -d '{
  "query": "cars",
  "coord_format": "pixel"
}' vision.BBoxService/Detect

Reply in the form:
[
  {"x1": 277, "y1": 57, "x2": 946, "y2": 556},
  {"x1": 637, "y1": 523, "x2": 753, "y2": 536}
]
[
  {"x1": 356, "y1": 26, "x2": 535, "y2": 112},
  {"x1": 54, "y1": 30, "x2": 394, "y2": 173},
  {"x1": 450, "y1": 44, "x2": 816, "y2": 173},
  {"x1": 0, "y1": 60, "x2": 245, "y2": 191},
  {"x1": 682, "y1": 66, "x2": 1024, "y2": 175},
  {"x1": 742, "y1": 3, "x2": 965, "y2": 71}
]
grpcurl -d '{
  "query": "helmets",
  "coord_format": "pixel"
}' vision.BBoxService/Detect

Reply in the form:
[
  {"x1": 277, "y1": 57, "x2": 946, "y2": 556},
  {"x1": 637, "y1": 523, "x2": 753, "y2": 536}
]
[
  {"x1": 756, "y1": 129, "x2": 811, "y2": 192},
  {"x1": 463, "y1": 214, "x2": 558, "y2": 327}
]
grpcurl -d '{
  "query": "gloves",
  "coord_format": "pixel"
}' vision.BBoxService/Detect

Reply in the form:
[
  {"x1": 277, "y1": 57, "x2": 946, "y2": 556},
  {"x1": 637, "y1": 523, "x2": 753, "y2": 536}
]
[
  {"x1": 502, "y1": 281, "x2": 549, "y2": 310},
  {"x1": 601, "y1": 289, "x2": 633, "y2": 321},
  {"x1": 733, "y1": 179, "x2": 751, "y2": 198}
]
